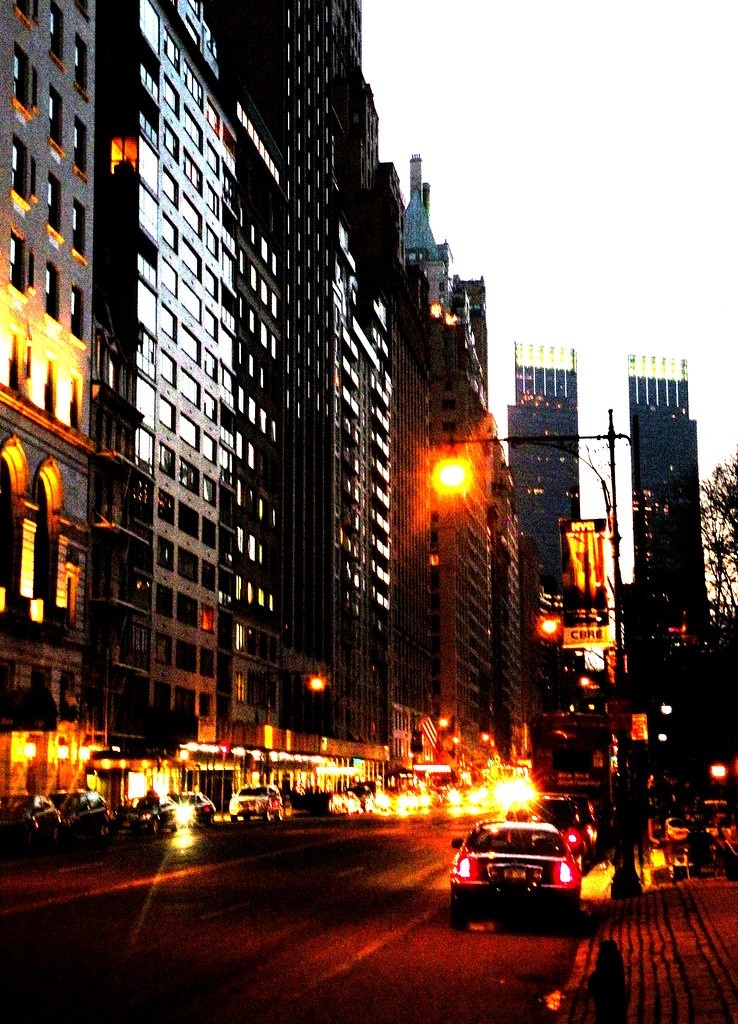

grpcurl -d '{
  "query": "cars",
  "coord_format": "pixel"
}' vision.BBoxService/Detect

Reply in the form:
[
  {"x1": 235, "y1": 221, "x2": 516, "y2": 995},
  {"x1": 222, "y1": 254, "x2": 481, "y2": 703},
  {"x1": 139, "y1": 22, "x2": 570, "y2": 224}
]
[
  {"x1": 228, "y1": 783, "x2": 283, "y2": 824},
  {"x1": 450, "y1": 822, "x2": 584, "y2": 939},
  {"x1": 507, "y1": 791, "x2": 602, "y2": 875},
  {"x1": 2, "y1": 789, "x2": 216, "y2": 860}
]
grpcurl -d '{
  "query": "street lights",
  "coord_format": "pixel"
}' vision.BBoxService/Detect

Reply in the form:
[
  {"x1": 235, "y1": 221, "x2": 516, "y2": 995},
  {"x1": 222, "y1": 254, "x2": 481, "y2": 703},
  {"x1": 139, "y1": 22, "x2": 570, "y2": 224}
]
[{"x1": 425, "y1": 408, "x2": 641, "y2": 904}]
